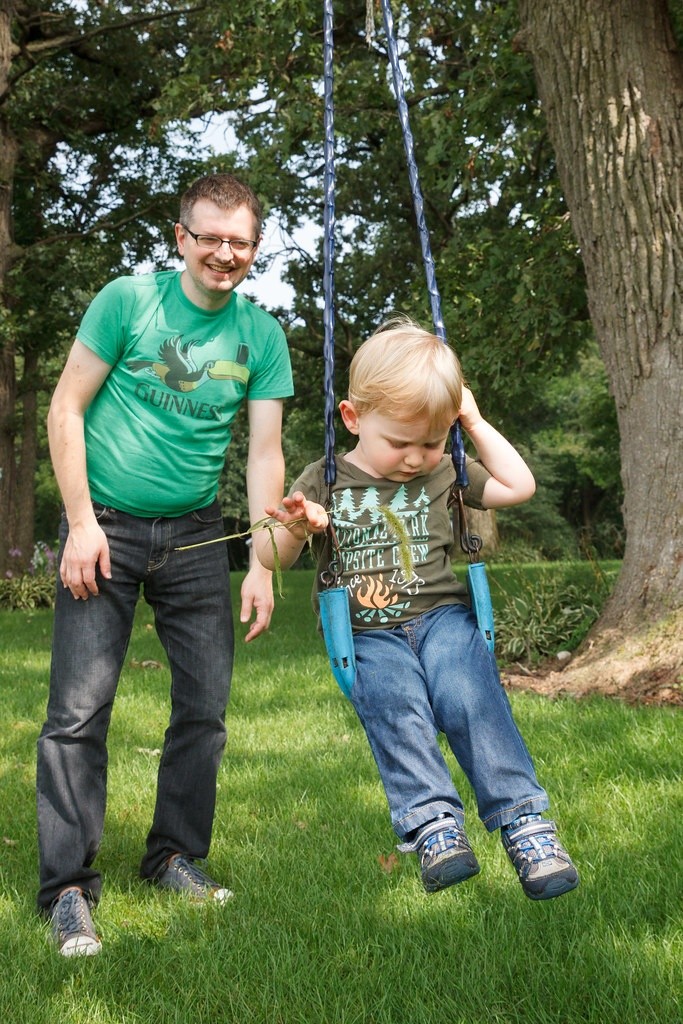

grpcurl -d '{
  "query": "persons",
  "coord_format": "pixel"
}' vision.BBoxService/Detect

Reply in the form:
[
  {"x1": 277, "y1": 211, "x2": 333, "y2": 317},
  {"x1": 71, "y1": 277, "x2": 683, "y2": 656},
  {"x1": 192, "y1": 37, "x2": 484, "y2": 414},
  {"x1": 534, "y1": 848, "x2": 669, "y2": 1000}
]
[
  {"x1": 255, "y1": 318, "x2": 580, "y2": 901},
  {"x1": 37, "y1": 175, "x2": 295, "y2": 958}
]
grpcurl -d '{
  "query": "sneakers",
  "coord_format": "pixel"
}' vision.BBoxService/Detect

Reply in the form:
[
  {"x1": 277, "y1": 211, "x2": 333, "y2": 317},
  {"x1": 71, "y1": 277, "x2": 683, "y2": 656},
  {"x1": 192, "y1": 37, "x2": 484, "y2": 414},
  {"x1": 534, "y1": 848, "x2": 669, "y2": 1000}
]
[
  {"x1": 151, "y1": 853, "x2": 233, "y2": 907},
  {"x1": 397, "y1": 813, "x2": 480, "y2": 892},
  {"x1": 50, "y1": 887, "x2": 102, "y2": 957},
  {"x1": 501, "y1": 814, "x2": 579, "y2": 900}
]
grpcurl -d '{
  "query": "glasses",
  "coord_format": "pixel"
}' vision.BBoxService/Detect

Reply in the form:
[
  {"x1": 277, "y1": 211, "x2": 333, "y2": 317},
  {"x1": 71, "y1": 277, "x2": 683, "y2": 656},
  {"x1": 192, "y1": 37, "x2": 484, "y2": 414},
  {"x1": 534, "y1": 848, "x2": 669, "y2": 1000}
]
[{"x1": 182, "y1": 223, "x2": 259, "y2": 253}]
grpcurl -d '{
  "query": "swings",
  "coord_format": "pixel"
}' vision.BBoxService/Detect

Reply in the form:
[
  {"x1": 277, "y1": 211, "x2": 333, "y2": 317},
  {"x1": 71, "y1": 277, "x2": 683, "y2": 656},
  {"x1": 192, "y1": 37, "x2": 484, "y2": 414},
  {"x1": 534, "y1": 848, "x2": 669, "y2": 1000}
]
[{"x1": 326, "y1": 0, "x2": 496, "y2": 705}]
